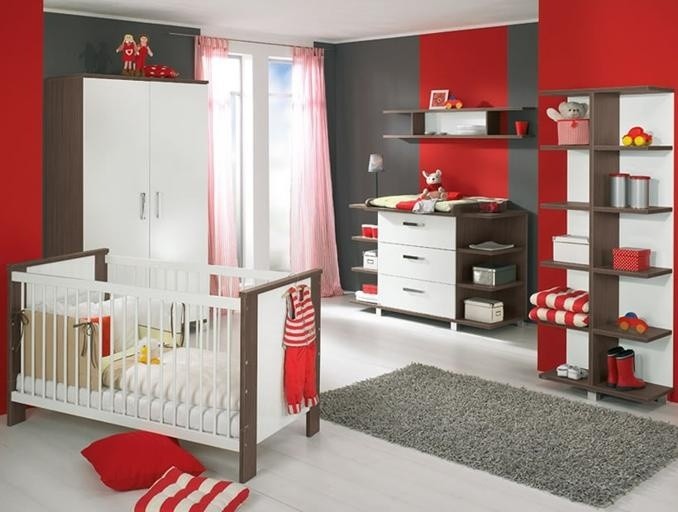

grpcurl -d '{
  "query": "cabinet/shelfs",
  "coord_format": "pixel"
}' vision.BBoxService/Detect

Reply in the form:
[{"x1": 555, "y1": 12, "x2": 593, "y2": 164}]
[
  {"x1": 345, "y1": 200, "x2": 530, "y2": 331},
  {"x1": 382, "y1": 107, "x2": 536, "y2": 140},
  {"x1": 43, "y1": 75, "x2": 213, "y2": 330},
  {"x1": 539, "y1": 83, "x2": 676, "y2": 407}
]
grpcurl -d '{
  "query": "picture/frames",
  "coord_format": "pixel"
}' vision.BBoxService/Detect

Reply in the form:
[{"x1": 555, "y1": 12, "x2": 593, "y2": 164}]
[{"x1": 428, "y1": 89, "x2": 450, "y2": 111}]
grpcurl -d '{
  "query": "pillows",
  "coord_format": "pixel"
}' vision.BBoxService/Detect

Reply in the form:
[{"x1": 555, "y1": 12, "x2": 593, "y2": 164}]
[
  {"x1": 69, "y1": 295, "x2": 138, "y2": 356},
  {"x1": 85, "y1": 315, "x2": 112, "y2": 358},
  {"x1": 132, "y1": 466, "x2": 249, "y2": 512},
  {"x1": 79, "y1": 429, "x2": 206, "y2": 493}
]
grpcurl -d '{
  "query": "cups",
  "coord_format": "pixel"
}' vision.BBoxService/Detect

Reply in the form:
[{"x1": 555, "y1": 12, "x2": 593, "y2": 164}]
[
  {"x1": 515, "y1": 121, "x2": 528, "y2": 137},
  {"x1": 629, "y1": 177, "x2": 650, "y2": 209},
  {"x1": 361, "y1": 225, "x2": 378, "y2": 239},
  {"x1": 608, "y1": 173, "x2": 629, "y2": 208}
]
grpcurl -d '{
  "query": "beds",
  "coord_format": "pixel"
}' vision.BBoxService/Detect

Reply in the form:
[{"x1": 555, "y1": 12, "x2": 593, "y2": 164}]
[{"x1": 6, "y1": 248, "x2": 323, "y2": 483}]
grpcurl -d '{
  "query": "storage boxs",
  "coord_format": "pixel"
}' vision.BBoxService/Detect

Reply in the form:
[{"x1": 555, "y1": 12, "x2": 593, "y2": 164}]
[
  {"x1": 470, "y1": 262, "x2": 518, "y2": 288},
  {"x1": 551, "y1": 232, "x2": 589, "y2": 265},
  {"x1": 611, "y1": 247, "x2": 651, "y2": 273},
  {"x1": 461, "y1": 296, "x2": 505, "y2": 325},
  {"x1": 358, "y1": 221, "x2": 378, "y2": 295}
]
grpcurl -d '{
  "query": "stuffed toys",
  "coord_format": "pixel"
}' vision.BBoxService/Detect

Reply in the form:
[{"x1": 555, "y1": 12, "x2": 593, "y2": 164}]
[
  {"x1": 134, "y1": 334, "x2": 164, "y2": 367},
  {"x1": 545, "y1": 98, "x2": 588, "y2": 123},
  {"x1": 415, "y1": 167, "x2": 448, "y2": 202}
]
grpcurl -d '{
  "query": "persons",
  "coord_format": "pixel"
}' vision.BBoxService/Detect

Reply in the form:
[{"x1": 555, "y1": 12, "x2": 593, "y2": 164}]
[
  {"x1": 113, "y1": 32, "x2": 139, "y2": 75},
  {"x1": 136, "y1": 33, "x2": 154, "y2": 75}
]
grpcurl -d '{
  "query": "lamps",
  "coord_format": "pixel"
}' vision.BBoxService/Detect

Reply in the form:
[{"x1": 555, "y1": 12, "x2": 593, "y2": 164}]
[{"x1": 366, "y1": 152, "x2": 385, "y2": 198}]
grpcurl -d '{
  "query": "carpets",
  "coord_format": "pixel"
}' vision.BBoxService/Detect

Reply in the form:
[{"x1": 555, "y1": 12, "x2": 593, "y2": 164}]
[{"x1": 315, "y1": 359, "x2": 677, "y2": 507}]
